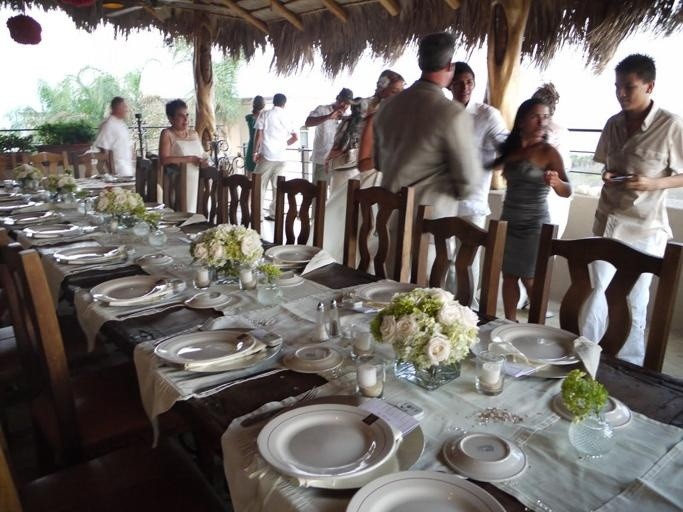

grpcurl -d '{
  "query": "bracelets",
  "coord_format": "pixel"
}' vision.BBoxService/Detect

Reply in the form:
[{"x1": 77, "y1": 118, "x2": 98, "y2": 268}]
[{"x1": 252, "y1": 151, "x2": 258, "y2": 156}]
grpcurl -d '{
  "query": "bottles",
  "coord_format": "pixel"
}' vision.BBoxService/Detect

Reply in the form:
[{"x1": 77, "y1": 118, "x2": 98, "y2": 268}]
[
  {"x1": 329, "y1": 298, "x2": 344, "y2": 338},
  {"x1": 313, "y1": 301, "x2": 327, "y2": 344}
]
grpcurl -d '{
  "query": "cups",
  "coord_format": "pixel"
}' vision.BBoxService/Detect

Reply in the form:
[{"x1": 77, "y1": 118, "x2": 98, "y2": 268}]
[
  {"x1": 474, "y1": 352, "x2": 504, "y2": 397},
  {"x1": 357, "y1": 354, "x2": 385, "y2": 397},
  {"x1": 350, "y1": 321, "x2": 374, "y2": 363}
]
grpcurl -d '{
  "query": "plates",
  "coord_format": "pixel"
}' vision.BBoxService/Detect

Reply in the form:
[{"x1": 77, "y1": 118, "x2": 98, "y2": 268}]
[
  {"x1": 181, "y1": 327, "x2": 281, "y2": 368},
  {"x1": 280, "y1": 343, "x2": 344, "y2": 373},
  {"x1": 255, "y1": 404, "x2": 392, "y2": 477},
  {"x1": 345, "y1": 470, "x2": 507, "y2": 512},
  {"x1": 551, "y1": 386, "x2": 632, "y2": 429},
  {"x1": 439, "y1": 434, "x2": 526, "y2": 482},
  {"x1": 469, "y1": 322, "x2": 588, "y2": 379},
  {"x1": 182, "y1": 294, "x2": 232, "y2": 311},
  {"x1": 357, "y1": 279, "x2": 414, "y2": 305},
  {"x1": 271, "y1": 396, "x2": 426, "y2": 486},
  {"x1": 265, "y1": 245, "x2": 320, "y2": 263},
  {"x1": 154, "y1": 331, "x2": 255, "y2": 362},
  {"x1": 0, "y1": 177, "x2": 173, "y2": 267},
  {"x1": 490, "y1": 323, "x2": 582, "y2": 364}
]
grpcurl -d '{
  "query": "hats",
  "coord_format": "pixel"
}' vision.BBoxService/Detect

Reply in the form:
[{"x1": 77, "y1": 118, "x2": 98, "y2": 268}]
[{"x1": 337, "y1": 87, "x2": 361, "y2": 105}]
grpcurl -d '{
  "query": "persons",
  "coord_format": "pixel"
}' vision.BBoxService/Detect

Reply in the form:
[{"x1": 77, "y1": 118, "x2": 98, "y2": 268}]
[
  {"x1": 577, "y1": 52, "x2": 683, "y2": 367},
  {"x1": 302, "y1": 30, "x2": 574, "y2": 322},
  {"x1": 94, "y1": 96, "x2": 135, "y2": 177},
  {"x1": 158, "y1": 97, "x2": 212, "y2": 217},
  {"x1": 249, "y1": 93, "x2": 300, "y2": 222},
  {"x1": 242, "y1": 93, "x2": 266, "y2": 224}
]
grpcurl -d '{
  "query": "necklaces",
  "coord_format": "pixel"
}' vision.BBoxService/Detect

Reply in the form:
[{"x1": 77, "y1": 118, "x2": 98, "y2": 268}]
[{"x1": 173, "y1": 128, "x2": 189, "y2": 141}]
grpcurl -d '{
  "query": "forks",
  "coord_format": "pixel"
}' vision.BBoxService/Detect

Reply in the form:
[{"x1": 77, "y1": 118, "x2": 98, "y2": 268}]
[{"x1": 241, "y1": 386, "x2": 319, "y2": 426}]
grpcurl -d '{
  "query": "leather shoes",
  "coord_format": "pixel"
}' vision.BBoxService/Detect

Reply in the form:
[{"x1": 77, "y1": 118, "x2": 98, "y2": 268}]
[{"x1": 264, "y1": 216, "x2": 276, "y2": 222}]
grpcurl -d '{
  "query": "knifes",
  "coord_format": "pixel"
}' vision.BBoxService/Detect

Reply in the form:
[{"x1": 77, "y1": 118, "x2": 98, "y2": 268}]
[{"x1": 115, "y1": 301, "x2": 182, "y2": 318}]
